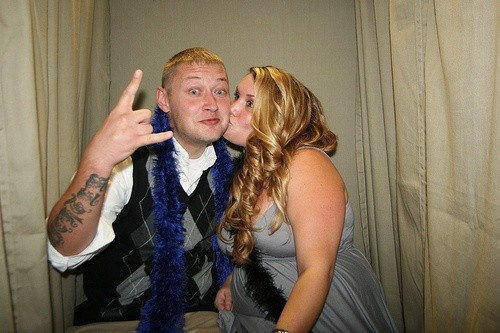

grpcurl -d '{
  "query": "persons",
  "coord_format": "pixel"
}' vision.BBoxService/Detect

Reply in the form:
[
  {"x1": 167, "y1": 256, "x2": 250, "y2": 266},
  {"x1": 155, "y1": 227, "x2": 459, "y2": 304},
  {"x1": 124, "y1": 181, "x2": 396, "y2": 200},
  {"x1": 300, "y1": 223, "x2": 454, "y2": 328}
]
[
  {"x1": 214, "y1": 65, "x2": 397, "y2": 333},
  {"x1": 47, "y1": 47, "x2": 236, "y2": 333}
]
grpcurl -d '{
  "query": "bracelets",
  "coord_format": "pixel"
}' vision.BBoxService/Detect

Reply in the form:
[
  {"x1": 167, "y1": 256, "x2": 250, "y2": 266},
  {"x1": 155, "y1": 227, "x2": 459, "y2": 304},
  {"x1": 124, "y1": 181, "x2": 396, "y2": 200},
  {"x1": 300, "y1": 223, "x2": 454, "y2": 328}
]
[{"x1": 271, "y1": 329, "x2": 290, "y2": 333}]
